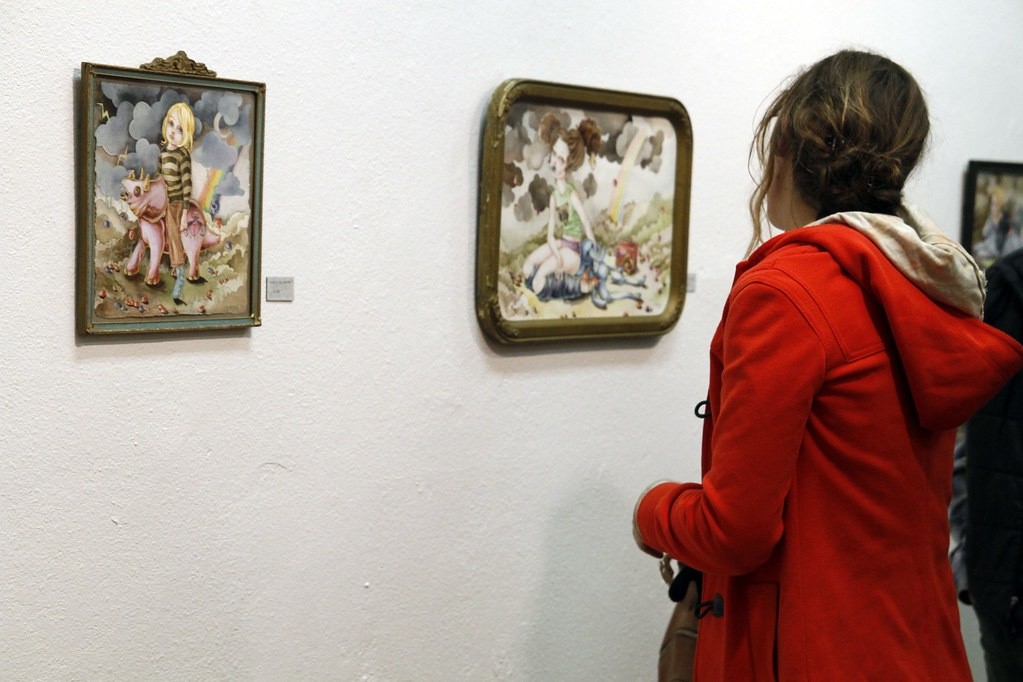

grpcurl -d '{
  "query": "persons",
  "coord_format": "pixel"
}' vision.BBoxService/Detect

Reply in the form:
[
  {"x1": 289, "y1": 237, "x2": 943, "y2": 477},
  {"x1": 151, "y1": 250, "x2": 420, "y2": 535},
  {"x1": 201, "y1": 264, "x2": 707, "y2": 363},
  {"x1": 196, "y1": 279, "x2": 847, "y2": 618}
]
[
  {"x1": 632, "y1": 52, "x2": 1023, "y2": 682},
  {"x1": 946, "y1": 248, "x2": 1023, "y2": 682}
]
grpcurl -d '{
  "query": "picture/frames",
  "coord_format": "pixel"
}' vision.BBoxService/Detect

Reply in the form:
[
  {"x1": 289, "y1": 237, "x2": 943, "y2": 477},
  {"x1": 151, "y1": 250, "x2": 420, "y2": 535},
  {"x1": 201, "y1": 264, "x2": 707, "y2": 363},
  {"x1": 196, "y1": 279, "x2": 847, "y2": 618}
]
[
  {"x1": 79, "y1": 51, "x2": 266, "y2": 335},
  {"x1": 961, "y1": 157, "x2": 1023, "y2": 272},
  {"x1": 473, "y1": 78, "x2": 689, "y2": 347}
]
